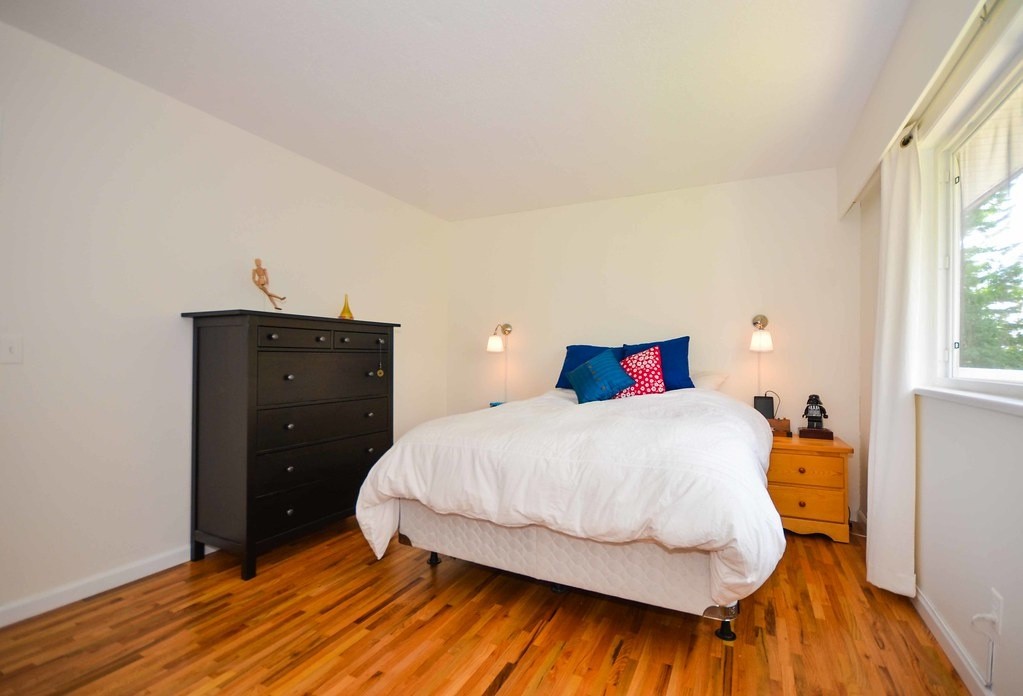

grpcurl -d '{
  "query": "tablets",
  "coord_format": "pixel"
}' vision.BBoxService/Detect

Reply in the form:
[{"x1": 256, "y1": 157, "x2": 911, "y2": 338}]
[{"x1": 753, "y1": 395, "x2": 774, "y2": 418}]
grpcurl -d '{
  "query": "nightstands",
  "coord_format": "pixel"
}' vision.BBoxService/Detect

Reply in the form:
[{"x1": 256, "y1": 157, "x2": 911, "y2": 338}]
[{"x1": 767, "y1": 437, "x2": 855, "y2": 544}]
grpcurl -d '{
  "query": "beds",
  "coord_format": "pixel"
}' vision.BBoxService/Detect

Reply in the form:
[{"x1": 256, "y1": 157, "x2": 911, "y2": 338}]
[{"x1": 356, "y1": 390, "x2": 785, "y2": 641}]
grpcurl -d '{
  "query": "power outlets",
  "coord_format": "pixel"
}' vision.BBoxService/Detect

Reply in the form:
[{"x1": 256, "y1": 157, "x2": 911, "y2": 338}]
[{"x1": 990, "y1": 587, "x2": 1003, "y2": 633}]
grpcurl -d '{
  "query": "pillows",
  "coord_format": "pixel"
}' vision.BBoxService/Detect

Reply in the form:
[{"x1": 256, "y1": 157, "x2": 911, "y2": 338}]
[
  {"x1": 612, "y1": 346, "x2": 665, "y2": 400},
  {"x1": 555, "y1": 344, "x2": 622, "y2": 390},
  {"x1": 623, "y1": 336, "x2": 695, "y2": 396},
  {"x1": 566, "y1": 349, "x2": 635, "y2": 404}
]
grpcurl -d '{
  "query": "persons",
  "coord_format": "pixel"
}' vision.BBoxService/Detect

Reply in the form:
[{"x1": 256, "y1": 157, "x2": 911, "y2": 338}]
[
  {"x1": 251, "y1": 259, "x2": 286, "y2": 310},
  {"x1": 803, "y1": 395, "x2": 828, "y2": 429}
]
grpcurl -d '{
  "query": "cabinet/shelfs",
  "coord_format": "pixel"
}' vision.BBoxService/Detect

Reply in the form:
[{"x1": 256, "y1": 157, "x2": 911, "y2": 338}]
[{"x1": 180, "y1": 308, "x2": 403, "y2": 583}]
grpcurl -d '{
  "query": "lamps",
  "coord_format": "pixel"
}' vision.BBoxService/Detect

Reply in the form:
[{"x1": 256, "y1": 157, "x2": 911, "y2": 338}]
[
  {"x1": 750, "y1": 314, "x2": 774, "y2": 354},
  {"x1": 487, "y1": 323, "x2": 513, "y2": 354}
]
